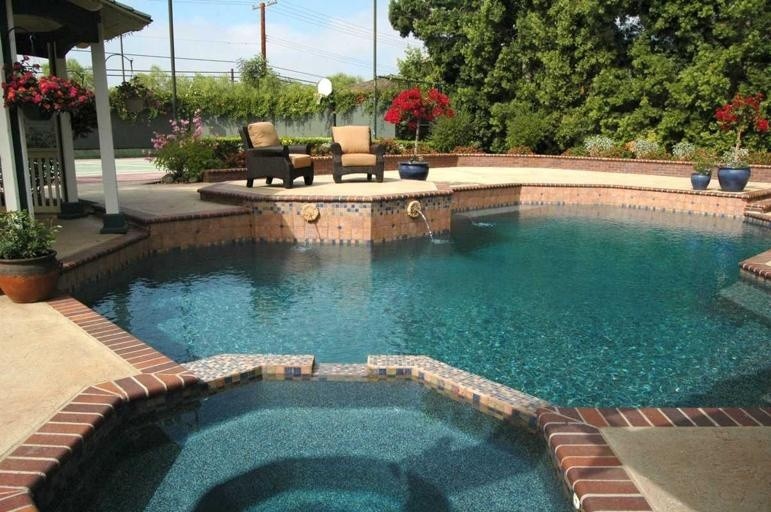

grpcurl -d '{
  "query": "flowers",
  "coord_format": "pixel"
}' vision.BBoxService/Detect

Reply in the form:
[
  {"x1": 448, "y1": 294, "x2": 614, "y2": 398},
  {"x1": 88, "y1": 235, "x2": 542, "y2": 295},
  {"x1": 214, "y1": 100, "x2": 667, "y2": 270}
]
[
  {"x1": 710, "y1": 90, "x2": 768, "y2": 169},
  {"x1": 383, "y1": 79, "x2": 455, "y2": 164},
  {"x1": 2, "y1": 54, "x2": 97, "y2": 111}
]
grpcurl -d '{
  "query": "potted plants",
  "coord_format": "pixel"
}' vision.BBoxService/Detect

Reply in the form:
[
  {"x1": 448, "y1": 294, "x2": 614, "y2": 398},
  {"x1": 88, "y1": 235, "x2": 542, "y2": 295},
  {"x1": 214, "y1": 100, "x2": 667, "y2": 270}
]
[
  {"x1": 398, "y1": 154, "x2": 430, "y2": 181},
  {"x1": 688, "y1": 158, "x2": 713, "y2": 191},
  {"x1": 0, "y1": 208, "x2": 69, "y2": 304}
]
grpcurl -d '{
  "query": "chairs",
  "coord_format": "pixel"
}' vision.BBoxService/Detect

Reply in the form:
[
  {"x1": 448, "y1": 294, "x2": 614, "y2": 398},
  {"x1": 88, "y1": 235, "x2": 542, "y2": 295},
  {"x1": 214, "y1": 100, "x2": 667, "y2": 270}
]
[
  {"x1": 328, "y1": 126, "x2": 386, "y2": 184},
  {"x1": 238, "y1": 119, "x2": 315, "y2": 189}
]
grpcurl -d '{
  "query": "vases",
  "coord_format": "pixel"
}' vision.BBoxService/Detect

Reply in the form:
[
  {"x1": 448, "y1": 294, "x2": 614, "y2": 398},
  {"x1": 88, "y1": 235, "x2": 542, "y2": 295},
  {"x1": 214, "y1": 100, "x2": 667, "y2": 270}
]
[
  {"x1": 717, "y1": 164, "x2": 751, "y2": 192},
  {"x1": 16, "y1": 100, "x2": 55, "y2": 121}
]
